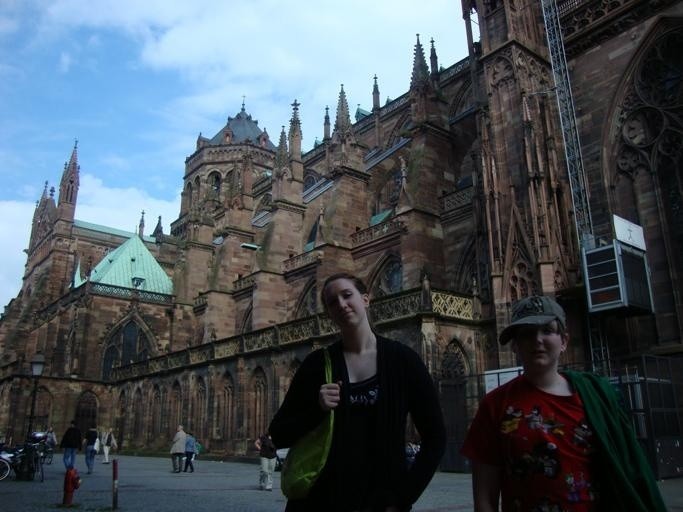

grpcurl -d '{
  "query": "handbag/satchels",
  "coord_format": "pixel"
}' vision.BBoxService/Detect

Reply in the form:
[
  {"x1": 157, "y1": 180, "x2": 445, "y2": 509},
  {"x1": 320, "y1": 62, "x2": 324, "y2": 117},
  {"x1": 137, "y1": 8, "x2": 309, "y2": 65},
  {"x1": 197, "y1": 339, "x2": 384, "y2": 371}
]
[{"x1": 280, "y1": 348, "x2": 335, "y2": 499}]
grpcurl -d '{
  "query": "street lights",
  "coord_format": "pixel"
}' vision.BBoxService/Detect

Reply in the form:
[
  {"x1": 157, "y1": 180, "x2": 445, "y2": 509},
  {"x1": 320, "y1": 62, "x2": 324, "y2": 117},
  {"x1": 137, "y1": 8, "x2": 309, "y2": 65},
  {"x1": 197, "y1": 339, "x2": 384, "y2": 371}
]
[{"x1": 14, "y1": 348, "x2": 50, "y2": 483}]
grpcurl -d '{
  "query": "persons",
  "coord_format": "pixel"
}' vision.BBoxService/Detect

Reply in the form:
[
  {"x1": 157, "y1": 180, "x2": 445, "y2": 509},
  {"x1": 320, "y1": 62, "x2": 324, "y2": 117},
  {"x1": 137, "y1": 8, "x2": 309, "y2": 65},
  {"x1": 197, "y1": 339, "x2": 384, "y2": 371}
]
[
  {"x1": 170, "y1": 425, "x2": 198, "y2": 474},
  {"x1": 44, "y1": 420, "x2": 118, "y2": 475},
  {"x1": 269, "y1": 274, "x2": 447, "y2": 512},
  {"x1": 254, "y1": 433, "x2": 277, "y2": 491},
  {"x1": 460, "y1": 296, "x2": 667, "y2": 512}
]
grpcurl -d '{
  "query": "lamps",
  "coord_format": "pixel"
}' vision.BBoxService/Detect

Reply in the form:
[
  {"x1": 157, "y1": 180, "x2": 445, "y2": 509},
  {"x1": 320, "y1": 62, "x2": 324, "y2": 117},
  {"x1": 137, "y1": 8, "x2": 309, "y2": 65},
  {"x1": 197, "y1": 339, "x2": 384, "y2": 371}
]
[{"x1": 240, "y1": 243, "x2": 258, "y2": 251}]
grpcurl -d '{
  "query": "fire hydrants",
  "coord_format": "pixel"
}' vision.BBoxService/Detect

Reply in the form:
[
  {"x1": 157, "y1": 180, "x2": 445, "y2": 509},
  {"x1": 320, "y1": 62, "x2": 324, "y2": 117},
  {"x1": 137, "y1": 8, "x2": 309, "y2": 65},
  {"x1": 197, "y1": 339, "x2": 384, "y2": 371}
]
[{"x1": 63, "y1": 464, "x2": 82, "y2": 507}]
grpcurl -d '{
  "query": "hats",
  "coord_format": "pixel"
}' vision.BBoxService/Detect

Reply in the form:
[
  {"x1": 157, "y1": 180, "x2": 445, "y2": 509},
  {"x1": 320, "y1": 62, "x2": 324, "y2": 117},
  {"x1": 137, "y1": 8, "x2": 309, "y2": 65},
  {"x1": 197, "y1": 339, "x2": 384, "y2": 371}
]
[{"x1": 499, "y1": 295, "x2": 567, "y2": 346}]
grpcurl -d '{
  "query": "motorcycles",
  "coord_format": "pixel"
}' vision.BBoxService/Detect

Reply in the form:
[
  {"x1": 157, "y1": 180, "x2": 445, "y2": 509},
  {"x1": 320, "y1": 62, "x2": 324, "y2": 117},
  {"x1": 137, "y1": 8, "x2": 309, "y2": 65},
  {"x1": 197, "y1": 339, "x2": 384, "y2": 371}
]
[{"x1": 0, "y1": 431, "x2": 50, "y2": 475}]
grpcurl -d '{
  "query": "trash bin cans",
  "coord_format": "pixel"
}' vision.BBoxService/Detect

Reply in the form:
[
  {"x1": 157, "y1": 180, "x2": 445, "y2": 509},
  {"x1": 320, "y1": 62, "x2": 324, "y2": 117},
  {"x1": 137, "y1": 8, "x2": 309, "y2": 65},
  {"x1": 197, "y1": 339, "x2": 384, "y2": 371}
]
[
  {"x1": 16, "y1": 451, "x2": 34, "y2": 481},
  {"x1": 232, "y1": 438, "x2": 247, "y2": 457}
]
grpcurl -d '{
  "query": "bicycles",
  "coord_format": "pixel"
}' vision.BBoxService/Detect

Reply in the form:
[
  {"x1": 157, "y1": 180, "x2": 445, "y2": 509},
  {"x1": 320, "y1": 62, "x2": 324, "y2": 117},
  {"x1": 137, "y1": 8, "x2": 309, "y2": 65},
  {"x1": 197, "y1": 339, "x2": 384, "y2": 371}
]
[{"x1": 0, "y1": 445, "x2": 57, "y2": 486}]
